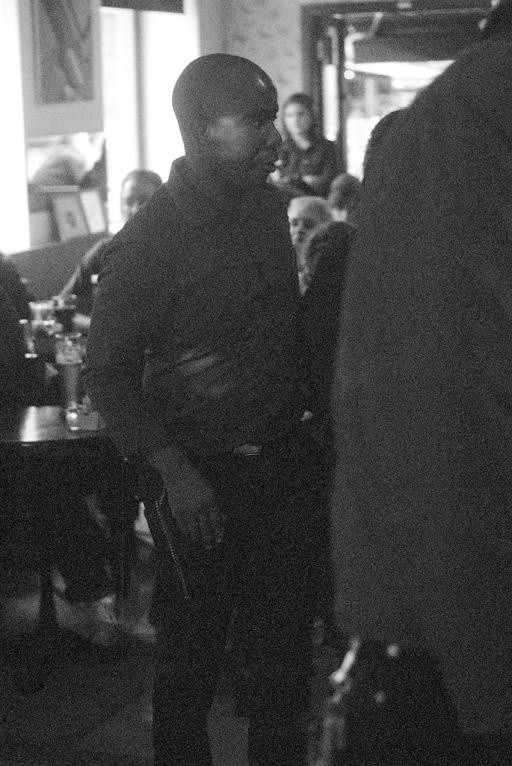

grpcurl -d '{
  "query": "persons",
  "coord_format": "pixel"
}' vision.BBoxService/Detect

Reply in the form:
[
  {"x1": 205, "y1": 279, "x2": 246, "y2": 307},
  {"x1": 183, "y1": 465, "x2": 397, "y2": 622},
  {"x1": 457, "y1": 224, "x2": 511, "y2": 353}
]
[
  {"x1": 311, "y1": 1, "x2": 510, "y2": 765},
  {"x1": 75, "y1": 52, "x2": 317, "y2": 766}
]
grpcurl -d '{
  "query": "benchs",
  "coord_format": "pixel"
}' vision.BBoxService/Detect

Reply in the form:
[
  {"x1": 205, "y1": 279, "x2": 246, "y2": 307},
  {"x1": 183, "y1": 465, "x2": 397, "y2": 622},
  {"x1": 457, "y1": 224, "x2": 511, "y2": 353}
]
[{"x1": 6, "y1": 223, "x2": 124, "y2": 301}]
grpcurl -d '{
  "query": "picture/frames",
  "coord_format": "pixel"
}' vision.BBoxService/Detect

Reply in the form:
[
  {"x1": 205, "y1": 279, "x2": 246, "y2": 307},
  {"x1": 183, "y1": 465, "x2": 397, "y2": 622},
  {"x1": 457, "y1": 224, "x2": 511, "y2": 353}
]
[{"x1": 43, "y1": 183, "x2": 90, "y2": 242}]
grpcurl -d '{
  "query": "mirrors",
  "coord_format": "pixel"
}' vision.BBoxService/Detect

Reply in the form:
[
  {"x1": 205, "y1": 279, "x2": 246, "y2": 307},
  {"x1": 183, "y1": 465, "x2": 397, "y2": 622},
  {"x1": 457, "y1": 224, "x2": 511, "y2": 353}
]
[{"x1": 26, "y1": 133, "x2": 109, "y2": 244}]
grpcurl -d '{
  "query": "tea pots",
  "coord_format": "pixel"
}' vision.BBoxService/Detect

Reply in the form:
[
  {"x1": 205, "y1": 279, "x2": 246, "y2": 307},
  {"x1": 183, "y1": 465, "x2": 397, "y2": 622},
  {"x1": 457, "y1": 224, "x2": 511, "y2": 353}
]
[{"x1": 316, "y1": 636, "x2": 457, "y2": 765}]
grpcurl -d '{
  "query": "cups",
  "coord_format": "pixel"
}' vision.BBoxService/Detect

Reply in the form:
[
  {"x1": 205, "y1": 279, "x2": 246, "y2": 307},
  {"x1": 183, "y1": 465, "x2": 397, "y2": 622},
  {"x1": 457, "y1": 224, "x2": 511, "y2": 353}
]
[{"x1": 23, "y1": 294, "x2": 91, "y2": 432}]
